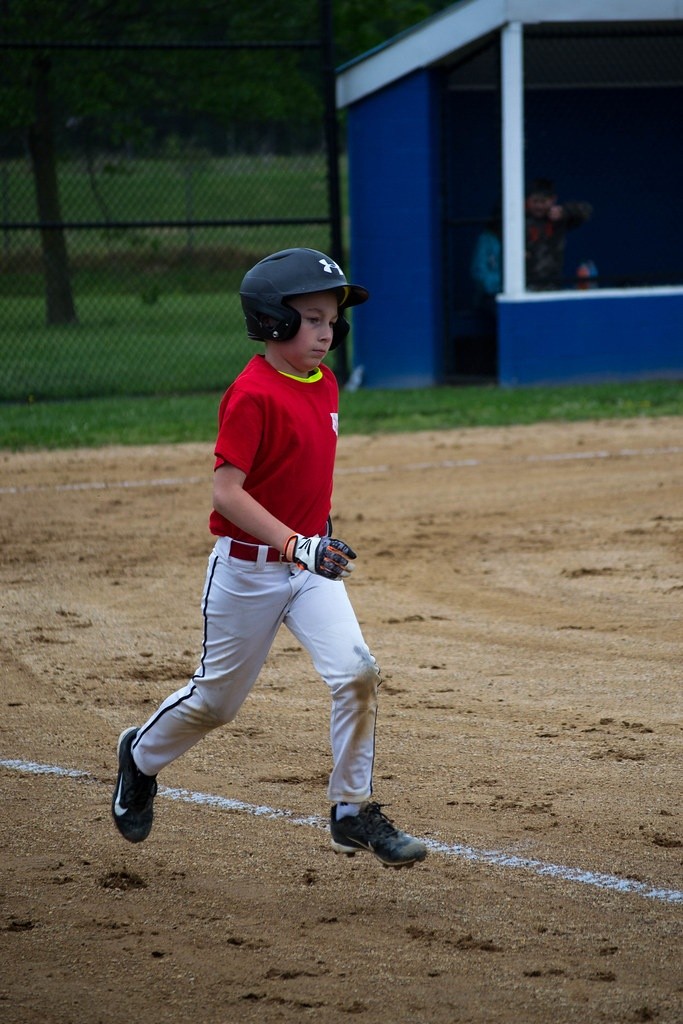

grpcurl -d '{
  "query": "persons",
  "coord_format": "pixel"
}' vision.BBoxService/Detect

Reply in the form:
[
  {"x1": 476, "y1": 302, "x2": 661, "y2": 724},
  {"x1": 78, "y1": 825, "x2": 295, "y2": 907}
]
[
  {"x1": 112, "y1": 246, "x2": 431, "y2": 871},
  {"x1": 472, "y1": 181, "x2": 605, "y2": 295}
]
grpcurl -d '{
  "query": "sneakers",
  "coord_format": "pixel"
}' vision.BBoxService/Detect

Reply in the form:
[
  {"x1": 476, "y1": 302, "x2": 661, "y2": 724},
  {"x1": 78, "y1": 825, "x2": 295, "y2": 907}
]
[
  {"x1": 330, "y1": 801, "x2": 428, "y2": 870},
  {"x1": 111, "y1": 727, "x2": 158, "y2": 843}
]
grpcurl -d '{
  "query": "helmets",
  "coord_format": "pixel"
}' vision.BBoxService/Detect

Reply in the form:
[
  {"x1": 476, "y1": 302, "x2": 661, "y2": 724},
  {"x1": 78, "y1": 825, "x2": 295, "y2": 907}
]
[{"x1": 239, "y1": 247, "x2": 369, "y2": 351}]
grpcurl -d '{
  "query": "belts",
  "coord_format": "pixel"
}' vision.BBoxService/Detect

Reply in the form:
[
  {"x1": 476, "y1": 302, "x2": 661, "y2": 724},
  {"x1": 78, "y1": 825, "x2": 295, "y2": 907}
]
[{"x1": 229, "y1": 541, "x2": 293, "y2": 564}]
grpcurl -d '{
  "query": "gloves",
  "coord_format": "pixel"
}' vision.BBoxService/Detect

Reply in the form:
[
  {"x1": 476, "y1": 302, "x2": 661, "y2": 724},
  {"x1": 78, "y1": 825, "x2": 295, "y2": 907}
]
[{"x1": 282, "y1": 532, "x2": 358, "y2": 582}]
